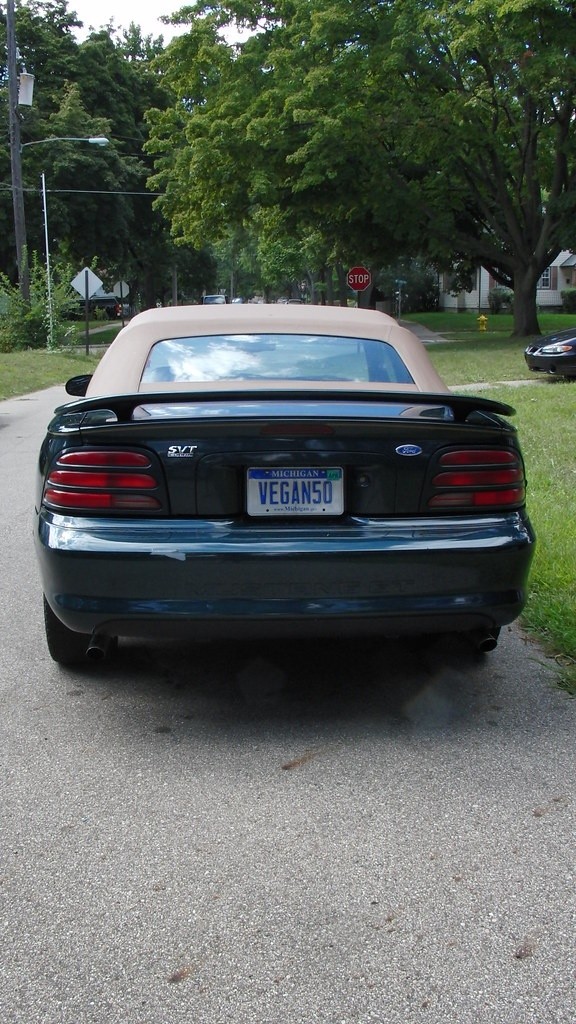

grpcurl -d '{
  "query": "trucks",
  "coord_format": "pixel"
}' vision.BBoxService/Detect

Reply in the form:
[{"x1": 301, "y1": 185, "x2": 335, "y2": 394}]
[{"x1": 201, "y1": 296, "x2": 228, "y2": 304}]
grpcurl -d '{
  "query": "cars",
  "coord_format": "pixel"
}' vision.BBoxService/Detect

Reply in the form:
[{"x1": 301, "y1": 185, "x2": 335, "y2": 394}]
[
  {"x1": 36, "y1": 303, "x2": 536, "y2": 673},
  {"x1": 525, "y1": 328, "x2": 576, "y2": 381}
]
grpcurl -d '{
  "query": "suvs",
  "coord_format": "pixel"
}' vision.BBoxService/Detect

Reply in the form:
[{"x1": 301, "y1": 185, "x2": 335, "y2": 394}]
[{"x1": 61, "y1": 297, "x2": 121, "y2": 320}]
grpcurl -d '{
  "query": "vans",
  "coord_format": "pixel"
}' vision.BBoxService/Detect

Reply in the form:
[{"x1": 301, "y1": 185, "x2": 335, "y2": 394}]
[{"x1": 285, "y1": 299, "x2": 301, "y2": 304}]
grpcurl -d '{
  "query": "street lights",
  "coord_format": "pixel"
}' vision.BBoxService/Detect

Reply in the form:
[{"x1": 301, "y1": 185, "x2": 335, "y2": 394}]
[
  {"x1": 10, "y1": 137, "x2": 110, "y2": 312},
  {"x1": 301, "y1": 283, "x2": 306, "y2": 302}
]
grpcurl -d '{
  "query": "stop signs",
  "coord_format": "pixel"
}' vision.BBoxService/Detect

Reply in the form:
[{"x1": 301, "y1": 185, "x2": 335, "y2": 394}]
[{"x1": 347, "y1": 267, "x2": 371, "y2": 292}]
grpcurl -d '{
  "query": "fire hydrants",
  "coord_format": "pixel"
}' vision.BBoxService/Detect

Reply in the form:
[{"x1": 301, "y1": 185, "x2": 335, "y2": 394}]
[{"x1": 476, "y1": 315, "x2": 488, "y2": 332}]
